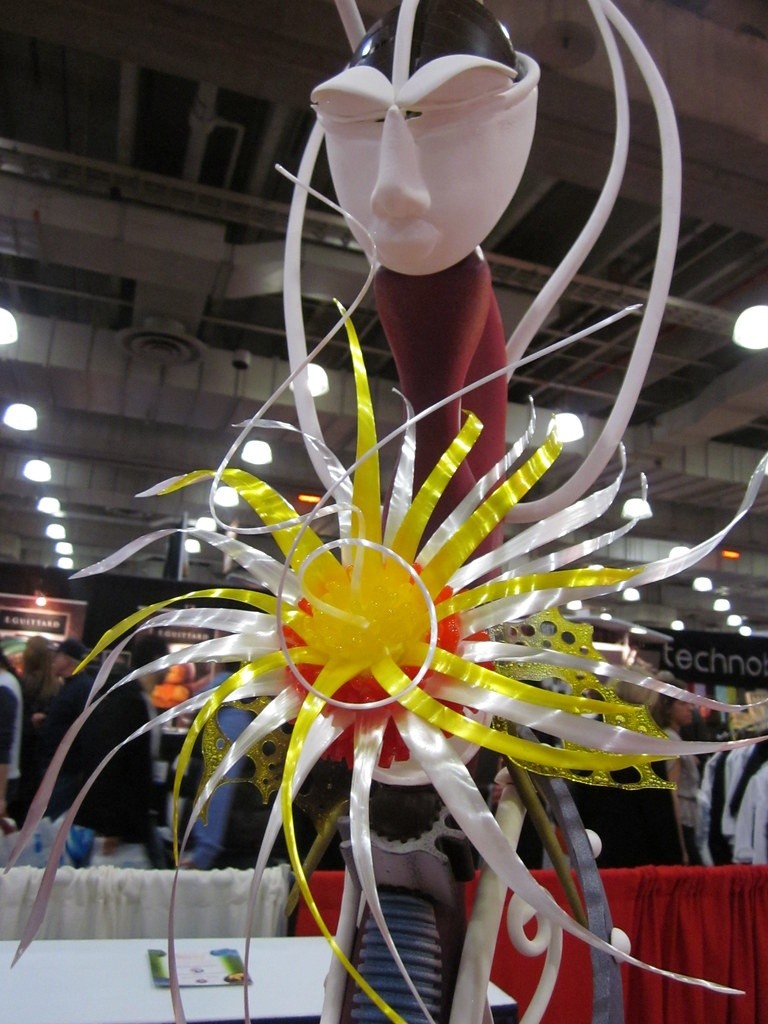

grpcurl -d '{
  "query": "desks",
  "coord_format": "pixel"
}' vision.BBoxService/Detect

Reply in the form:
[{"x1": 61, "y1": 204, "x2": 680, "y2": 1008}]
[{"x1": 0, "y1": 937, "x2": 520, "y2": 1024}]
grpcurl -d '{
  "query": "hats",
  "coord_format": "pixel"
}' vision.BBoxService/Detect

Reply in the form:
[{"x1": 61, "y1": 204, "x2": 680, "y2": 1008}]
[{"x1": 48, "y1": 638, "x2": 86, "y2": 661}]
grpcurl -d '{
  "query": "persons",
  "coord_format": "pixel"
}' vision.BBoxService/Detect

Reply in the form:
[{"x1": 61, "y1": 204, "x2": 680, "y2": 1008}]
[{"x1": 1, "y1": 629, "x2": 702, "y2": 870}]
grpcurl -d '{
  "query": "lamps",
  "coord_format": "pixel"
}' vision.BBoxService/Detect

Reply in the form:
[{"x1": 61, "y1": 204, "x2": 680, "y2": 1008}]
[{"x1": 0, "y1": 300, "x2": 768, "y2": 637}]
